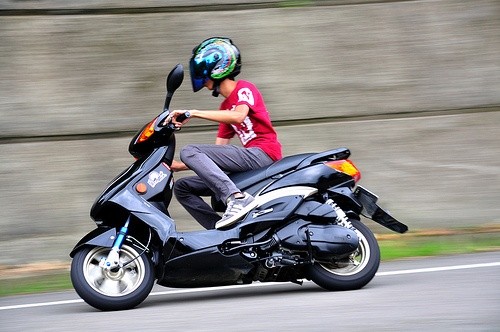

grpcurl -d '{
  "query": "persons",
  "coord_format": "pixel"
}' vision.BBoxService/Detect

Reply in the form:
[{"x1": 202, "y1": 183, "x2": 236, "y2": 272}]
[{"x1": 162, "y1": 35, "x2": 283, "y2": 231}]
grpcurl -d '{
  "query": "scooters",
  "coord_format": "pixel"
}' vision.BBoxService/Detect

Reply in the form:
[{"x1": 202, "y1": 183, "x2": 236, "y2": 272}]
[{"x1": 70, "y1": 64, "x2": 408, "y2": 311}]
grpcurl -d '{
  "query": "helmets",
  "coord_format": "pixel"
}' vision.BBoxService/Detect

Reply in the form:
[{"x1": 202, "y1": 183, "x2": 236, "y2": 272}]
[{"x1": 190, "y1": 37, "x2": 242, "y2": 96}]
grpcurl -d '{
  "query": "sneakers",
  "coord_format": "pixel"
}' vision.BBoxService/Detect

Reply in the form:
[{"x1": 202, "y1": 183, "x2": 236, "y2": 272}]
[{"x1": 214, "y1": 190, "x2": 258, "y2": 230}]
